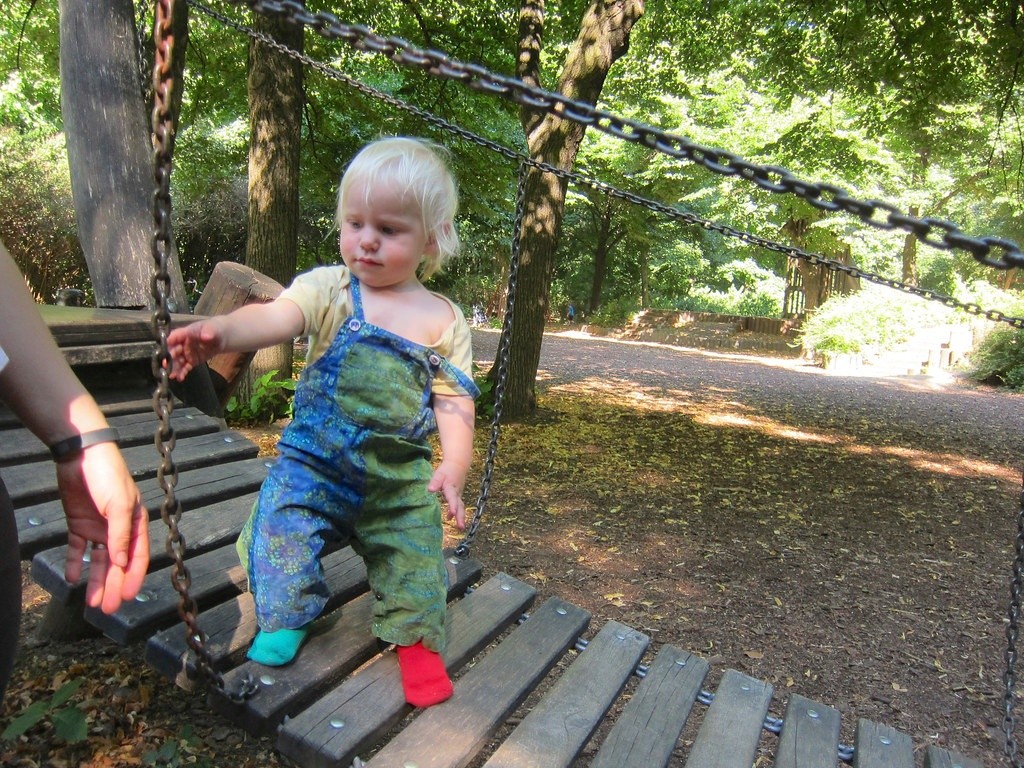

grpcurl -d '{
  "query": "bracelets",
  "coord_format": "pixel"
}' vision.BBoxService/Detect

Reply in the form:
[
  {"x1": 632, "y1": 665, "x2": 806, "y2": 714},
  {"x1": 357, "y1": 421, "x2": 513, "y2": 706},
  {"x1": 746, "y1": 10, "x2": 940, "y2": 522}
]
[{"x1": 50, "y1": 427, "x2": 121, "y2": 463}]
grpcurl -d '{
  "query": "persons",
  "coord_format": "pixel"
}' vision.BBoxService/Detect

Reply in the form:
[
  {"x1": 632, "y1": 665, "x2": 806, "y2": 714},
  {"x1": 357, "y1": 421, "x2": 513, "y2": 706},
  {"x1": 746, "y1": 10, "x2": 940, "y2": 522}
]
[
  {"x1": 567, "y1": 302, "x2": 579, "y2": 327},
  {"x1": 0, "y1": 237, "x2": 152, "y2": 712},
  {"x1": 162, "y1": 137, "x2": 483, "y2": 708}
]
